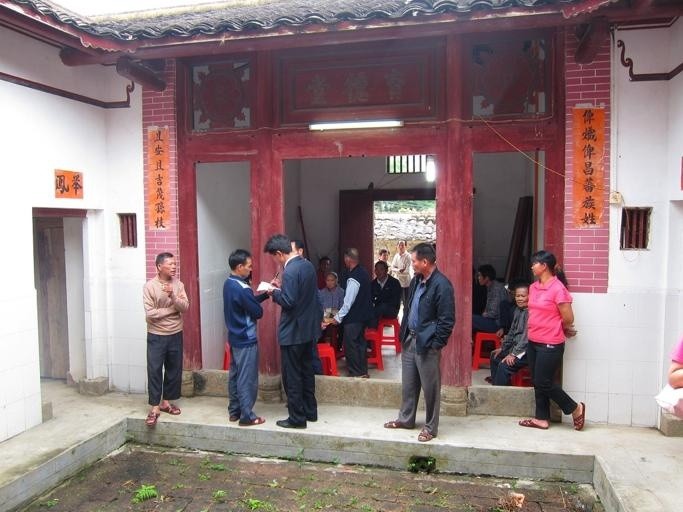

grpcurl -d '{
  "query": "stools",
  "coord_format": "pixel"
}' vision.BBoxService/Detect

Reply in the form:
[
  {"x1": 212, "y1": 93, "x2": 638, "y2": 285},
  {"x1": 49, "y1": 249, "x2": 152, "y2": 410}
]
[
  {"x1": 224, "y1": 343, "x2": 231, "y2": 371},
  {"x1": 318, "y1": 325, "x2": 346, "y2": 376},
  {"x1": 473, "y1": 332, "x2": 501, "y2": 371},
  {"x1": 376, "y1": 316, "x2": 400, "y2": 355},
  {"x1": 512, "y1": 369, "x2": 533, "y2": 387},
  {"x1": 362, "y1": 329, "x2": 383, "y2": 370}
]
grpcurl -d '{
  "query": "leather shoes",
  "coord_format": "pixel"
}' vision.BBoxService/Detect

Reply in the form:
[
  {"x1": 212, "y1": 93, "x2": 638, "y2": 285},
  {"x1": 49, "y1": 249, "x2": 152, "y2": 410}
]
[{"x1": 277, "y1": 417, "x2": 306, "y2": 429}]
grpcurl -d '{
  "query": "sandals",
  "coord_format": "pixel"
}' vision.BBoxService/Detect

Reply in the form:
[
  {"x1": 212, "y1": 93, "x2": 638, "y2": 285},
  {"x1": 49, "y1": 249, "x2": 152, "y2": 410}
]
[
  {"x1": 238, "y1": 416, "x2": 265, "y2": 426},
  {"x1": 384, "y1": 419, "x2": 405, "y2": 428},
  {"x1": 418, "y1": 427, "x2": 434, "y2": 441}
]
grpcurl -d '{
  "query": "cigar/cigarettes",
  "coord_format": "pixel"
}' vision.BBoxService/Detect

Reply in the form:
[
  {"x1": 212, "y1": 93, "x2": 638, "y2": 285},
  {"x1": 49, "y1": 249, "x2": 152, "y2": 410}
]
[{"x1": 162, "y1": 285, "x2": 165, "y2": 288}]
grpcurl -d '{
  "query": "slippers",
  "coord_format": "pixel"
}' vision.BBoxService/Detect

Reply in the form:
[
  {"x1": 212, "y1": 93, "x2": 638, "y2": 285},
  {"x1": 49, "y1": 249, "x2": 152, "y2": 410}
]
[
  {"x1": 519, "y1": 420, "x2": 548, "y2": 429},
  {"x1": 573, "y1": 401, "x2": 585, "y2": 430},
  {"x1": 145, "y1": 412, "x2": 160, "y2": 426},
  {"x1": 160, "y1": 404, "x2": 182, "y2": 415}
]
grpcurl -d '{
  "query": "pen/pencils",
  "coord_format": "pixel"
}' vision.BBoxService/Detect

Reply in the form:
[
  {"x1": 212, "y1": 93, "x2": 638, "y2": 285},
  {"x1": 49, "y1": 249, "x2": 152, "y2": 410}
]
[{"x1": 271, "y1": 272, "x2": 280, "y2": 285}]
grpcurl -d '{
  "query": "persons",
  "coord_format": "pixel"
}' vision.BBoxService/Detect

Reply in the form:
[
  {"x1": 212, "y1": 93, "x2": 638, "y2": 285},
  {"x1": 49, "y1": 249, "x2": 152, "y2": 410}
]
[
  {"x1": 142, "y1": 252, "x2": 189, "y2": 428},
  {"x1": 281, "y1": 239, "x2": 309, "y2": 408},
  {"x1": 224, "y1": 248, "x2": 273, "y2": 426},
  {"x1": 472, "y1": 264, "x2": 508, "y2": 334},
  {"x1": 384, "y1": 243, "x2": 455, "y2": 441},
  {"x1": 518, "y1": 250, "x2": 585, "y2": 431},
  {"x1": 668, "y1": 334, "x2": 683, "y2": 389},
  {"x1": 263, "y1": 234, "x2": 318, "y2": 428},
  {"x1": 367, "y1": 238, "x2": 415, "y2": 349},
  {"x1": 316, "y1": 256, "x2": 345, "y2": 353},
  {"x1": 331, "y1": 247, "x2": 370, "y2": 379},
  {"x1": 485, "y1": 285, "x2": 530, "y2": 386}
]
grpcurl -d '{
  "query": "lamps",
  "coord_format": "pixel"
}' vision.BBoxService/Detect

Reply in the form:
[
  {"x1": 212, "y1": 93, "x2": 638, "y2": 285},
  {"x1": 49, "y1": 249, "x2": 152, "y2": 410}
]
[{"x1": 309, "y1": 120, "x2": 404, "y2": 130}]
